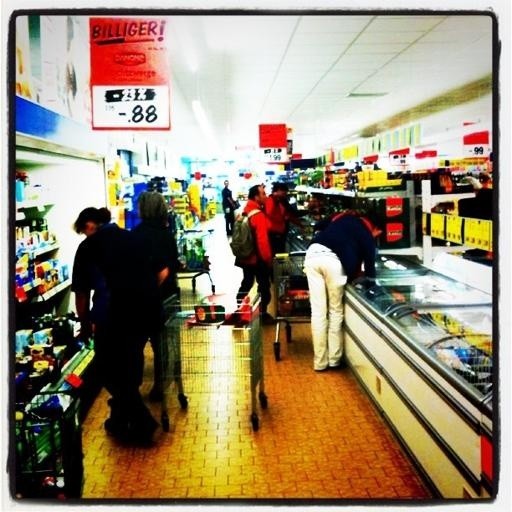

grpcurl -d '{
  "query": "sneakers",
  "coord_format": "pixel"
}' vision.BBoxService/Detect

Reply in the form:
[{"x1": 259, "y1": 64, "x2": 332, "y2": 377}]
[
  {"x1": 107, "y1": 397, "x2": 114, "y2": 407},
  {"x1": 103, "y1": 412, "x2": 159, "y2": 449},
  {"x1": 148, "y1": 382, "x2": 167, "y2": 403},
  {"x1": 227, "y1": 230, "x2": 235, "y2": 236},
  {"x1": 260, "y1": 312, "x2": 278, "y2": 326}
]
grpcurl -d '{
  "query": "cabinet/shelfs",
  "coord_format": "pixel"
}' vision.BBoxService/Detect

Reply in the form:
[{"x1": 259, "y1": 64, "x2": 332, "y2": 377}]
[
  {"x1": 414, "y1": 177, "x2": 479, "y2": 256},
  {"x1": 291, "y1": 175, "x2": 421, "y2": 258},
  {"x1": 12, "y1": 144, "x2": 103, "y2": 427}
]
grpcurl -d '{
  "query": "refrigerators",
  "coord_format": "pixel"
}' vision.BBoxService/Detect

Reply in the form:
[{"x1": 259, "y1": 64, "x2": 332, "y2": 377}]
[{"x1": 342, "y1": 258, "x2": 496, "y2": 500}]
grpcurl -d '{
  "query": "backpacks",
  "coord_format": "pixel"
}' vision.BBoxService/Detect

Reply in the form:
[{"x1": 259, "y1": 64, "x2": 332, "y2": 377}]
[{"x1": 229, "y1": 208, "x2": 260, "y2": 262}]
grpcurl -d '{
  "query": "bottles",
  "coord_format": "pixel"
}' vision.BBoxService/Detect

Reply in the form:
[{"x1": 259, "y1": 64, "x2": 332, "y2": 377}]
[
  {"x1": 324, "y1": 176, "x2": 331, "y2": 189},
  {"x1": 15, "y1": 171, "x2": 29, "y2": 202},
  {"x1": 14, "y1": 411, "x2": 28, "y2": 469}
]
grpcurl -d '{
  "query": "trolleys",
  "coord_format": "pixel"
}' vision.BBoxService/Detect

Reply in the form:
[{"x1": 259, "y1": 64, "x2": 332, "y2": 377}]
[
  {"x1": 174, "y1": 226, "x2": 216, "y2": 297},
  {"x1": 154, "y1": 283, "x2": 274, "y2": 435},
  {"x1": 266, "y1": 248, "x2": 312, "y2": 360},
  {"x1": 11, "y1": 373, "x2": 88, "y2": 500}
]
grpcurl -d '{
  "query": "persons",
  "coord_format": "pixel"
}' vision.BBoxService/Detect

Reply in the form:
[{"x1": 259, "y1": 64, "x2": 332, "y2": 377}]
[
  {"x1": 132, "y1": 191, "x2": 181, "y2": 400},
  {"x1": 263, "y1": 180, "x2": 309, "y2": 285},
  {"x1": 231, "y1": 181, "x2": 280, "y2": 327},
  {"x1": 70, "y1": 207, "x2": 168, "y2": 449},
  {"x1": 222, "y1": 180, "x2": 239, "y2": 238},
  {"x1": 304, "y1": 206, "x2": 383, "y2": 371}
]
